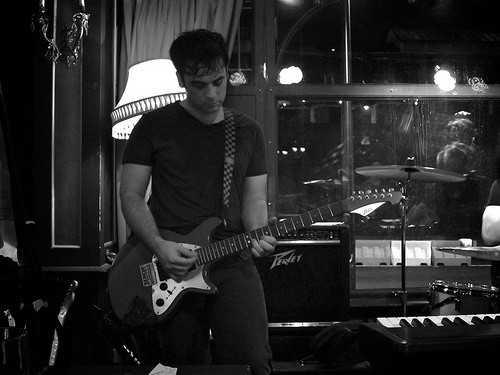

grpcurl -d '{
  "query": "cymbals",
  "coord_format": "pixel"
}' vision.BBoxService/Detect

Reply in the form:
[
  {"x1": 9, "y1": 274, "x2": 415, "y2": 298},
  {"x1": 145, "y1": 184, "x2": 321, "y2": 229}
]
[{"x1": 354, "y1": 165, "x2": 467, "y2": 183}]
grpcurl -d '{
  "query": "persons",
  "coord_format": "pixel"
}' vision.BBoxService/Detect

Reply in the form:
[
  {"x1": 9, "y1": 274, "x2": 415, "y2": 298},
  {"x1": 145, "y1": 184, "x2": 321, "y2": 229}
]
[
  {"x1": 482, "y1": 177, "x2": 500, "y2": 288},
  {"x1": 407, "y1": 147, "x2": 485, "y2": 247},
  {"x1": 120, "y1": 29, "x2": 278, "y2": 375}
]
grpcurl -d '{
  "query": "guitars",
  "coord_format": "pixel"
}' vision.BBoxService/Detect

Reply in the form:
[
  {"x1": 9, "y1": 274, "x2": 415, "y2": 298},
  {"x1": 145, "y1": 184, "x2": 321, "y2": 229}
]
[{"x1": 107, "y1": 187, "x2": 402, "y2": 327}]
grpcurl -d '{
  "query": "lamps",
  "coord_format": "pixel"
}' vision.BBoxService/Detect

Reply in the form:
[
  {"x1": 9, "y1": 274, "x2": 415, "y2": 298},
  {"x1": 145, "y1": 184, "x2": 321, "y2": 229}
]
[{"x1": 108, "y1": 57, "x2": 187, "y2": 142}]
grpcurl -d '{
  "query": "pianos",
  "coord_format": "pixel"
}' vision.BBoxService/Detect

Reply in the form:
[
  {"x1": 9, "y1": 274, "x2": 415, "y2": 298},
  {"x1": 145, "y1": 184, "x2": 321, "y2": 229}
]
[{"x1": 357, "y1": 312, "x2": 500, "y2": 375}]
